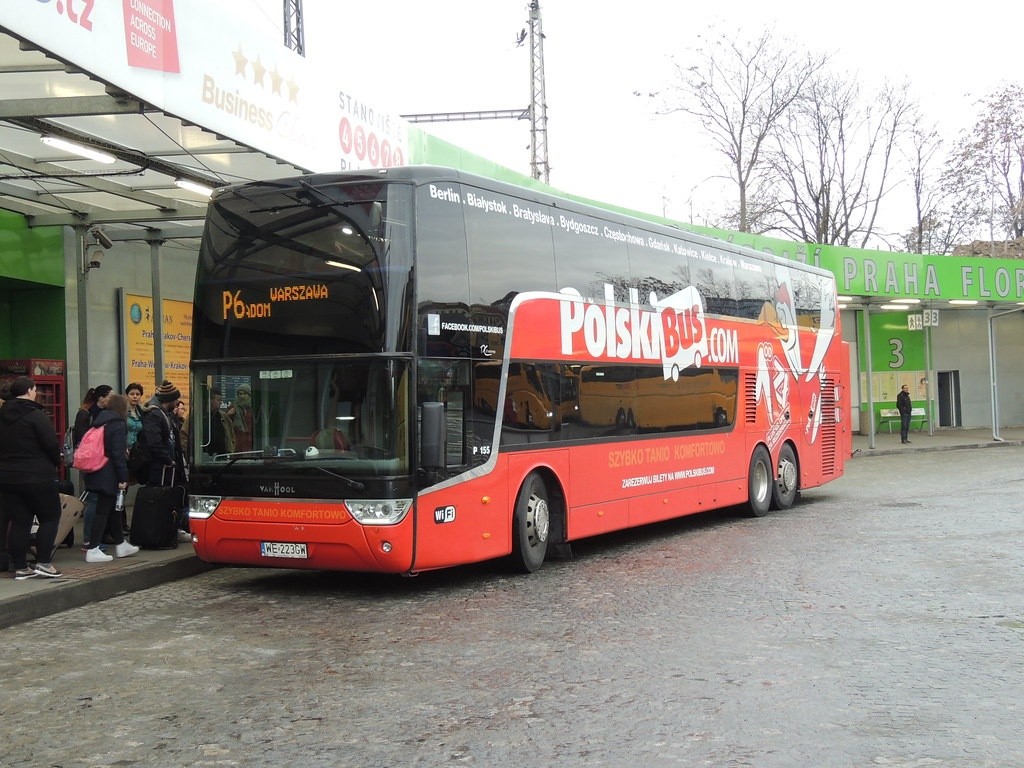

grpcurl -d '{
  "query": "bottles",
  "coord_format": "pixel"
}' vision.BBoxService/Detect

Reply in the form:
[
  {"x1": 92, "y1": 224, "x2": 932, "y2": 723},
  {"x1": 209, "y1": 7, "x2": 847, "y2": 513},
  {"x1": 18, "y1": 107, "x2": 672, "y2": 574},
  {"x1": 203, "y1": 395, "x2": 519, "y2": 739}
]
[{"x1": 115, "y1": 486, "x2": 127, "y2": 512}]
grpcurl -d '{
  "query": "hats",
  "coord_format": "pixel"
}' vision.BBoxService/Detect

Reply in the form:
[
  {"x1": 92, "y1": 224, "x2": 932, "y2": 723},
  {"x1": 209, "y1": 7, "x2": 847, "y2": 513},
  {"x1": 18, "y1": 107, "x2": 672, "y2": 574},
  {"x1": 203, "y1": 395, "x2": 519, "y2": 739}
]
[
  {"x1": 159, "y1": 380, "x2": 180, "y2": 401},
  {"x1": 239, "y1": 383, "x2": 251, "y2": 396}
]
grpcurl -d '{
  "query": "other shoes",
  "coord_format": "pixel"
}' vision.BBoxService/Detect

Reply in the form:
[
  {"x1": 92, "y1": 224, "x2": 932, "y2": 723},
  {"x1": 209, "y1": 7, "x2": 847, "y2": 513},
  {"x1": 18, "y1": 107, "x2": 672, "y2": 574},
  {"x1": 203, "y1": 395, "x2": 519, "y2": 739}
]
[
  {"x1": 902, "y1": 440, "x2": 911, "y2": 444},
  {"x1": 82, "y1": 540, "x2": 108, "y2": 551}
]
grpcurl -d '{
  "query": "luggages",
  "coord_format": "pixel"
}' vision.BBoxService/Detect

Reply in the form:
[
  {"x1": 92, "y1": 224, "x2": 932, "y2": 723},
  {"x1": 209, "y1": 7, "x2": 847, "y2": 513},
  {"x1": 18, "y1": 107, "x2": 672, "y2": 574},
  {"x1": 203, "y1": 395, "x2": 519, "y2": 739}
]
[
  {"x1": 26, "y1": 490, "x2": 90, "y2": 560},
  {"x1": 129, "y1": 464, "x2": 185, "y2": 551}
]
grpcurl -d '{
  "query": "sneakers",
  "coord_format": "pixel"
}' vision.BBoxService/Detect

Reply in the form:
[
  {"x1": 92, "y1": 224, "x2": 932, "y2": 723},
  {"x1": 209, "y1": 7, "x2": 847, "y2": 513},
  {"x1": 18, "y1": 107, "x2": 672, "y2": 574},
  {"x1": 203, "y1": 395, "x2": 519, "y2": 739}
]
[
  {"x1": 116, "y1": 539, "x2": 140, "y2": 557},
  {"x1": 86, "y1": 546, "x2": 114, "y2": 562},
  {"x1": 176, "y1": 528, "x2": 192, "y2": 542},
  {"x1": 15, "y1": 562, "x2": 39, "y2": 580},
  {"x1": 33, "y1": 563, "x2": 62, "y2": 577}
]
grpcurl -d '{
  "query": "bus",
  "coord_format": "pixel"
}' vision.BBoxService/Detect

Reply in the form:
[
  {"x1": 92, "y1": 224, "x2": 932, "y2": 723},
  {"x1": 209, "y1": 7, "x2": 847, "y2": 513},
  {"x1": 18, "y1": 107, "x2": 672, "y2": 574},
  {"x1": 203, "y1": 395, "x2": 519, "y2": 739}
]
[
  {"x1": 187, "y1": 165, "x2": 853, "y2": 577},
  {"x1": 474, "y1": 359, "x2": 579, "y2": 429},
  {"x1": 576, "y1": 364, "x2": 740, "y2": 429}
]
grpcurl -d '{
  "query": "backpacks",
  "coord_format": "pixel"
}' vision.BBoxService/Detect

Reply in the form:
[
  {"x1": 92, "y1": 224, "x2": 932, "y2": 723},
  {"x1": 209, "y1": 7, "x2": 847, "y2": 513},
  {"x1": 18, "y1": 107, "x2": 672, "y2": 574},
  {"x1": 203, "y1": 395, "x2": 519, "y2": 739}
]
[{"x1": 73, "y1": 422, "x2": 109, "y2": 472}]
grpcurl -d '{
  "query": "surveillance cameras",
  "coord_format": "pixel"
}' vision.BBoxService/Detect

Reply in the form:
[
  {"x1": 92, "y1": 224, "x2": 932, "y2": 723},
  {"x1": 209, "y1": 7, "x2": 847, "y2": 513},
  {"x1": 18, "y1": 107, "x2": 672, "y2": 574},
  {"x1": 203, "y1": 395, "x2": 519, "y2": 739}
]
[
  {"x1": 90, "y1": 251, "x2": 105, "y2": 267},
  {"x1": 92, "y1": 227, "x2": 113, "y2": 250}
]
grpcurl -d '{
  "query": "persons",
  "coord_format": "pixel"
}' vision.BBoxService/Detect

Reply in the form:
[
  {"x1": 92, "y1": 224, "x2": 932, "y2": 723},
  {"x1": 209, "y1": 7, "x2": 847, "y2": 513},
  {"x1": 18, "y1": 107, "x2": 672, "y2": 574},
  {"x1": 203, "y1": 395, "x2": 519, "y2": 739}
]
[
  {"x1": 226, "y1": 383, "x2": 254, "y2": 454},
  {"x1": 179, "y1": 388, "x2": 235, "y2": 461},
  {"x1": 0, "y1": 375, "x2": 191, "y2": 580},
  {"x1": 897, "y1": 385, "x2": 912, "y2": 444}
]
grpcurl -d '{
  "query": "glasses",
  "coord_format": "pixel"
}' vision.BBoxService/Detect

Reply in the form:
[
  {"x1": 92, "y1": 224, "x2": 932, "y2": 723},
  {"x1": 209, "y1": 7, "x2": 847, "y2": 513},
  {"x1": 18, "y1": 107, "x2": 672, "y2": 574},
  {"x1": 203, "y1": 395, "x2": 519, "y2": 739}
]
[{"x1": 179, "y1": 407, "x2": 186, "y2": 412}]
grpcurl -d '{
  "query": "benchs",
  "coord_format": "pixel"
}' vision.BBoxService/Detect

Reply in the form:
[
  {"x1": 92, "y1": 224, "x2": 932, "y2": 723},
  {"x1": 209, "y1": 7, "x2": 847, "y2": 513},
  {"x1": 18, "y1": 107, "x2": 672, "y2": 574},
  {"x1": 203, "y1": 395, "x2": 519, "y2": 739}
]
[{"x1": 877, "y1": 408, "x2": 934, "y2": 434}]
